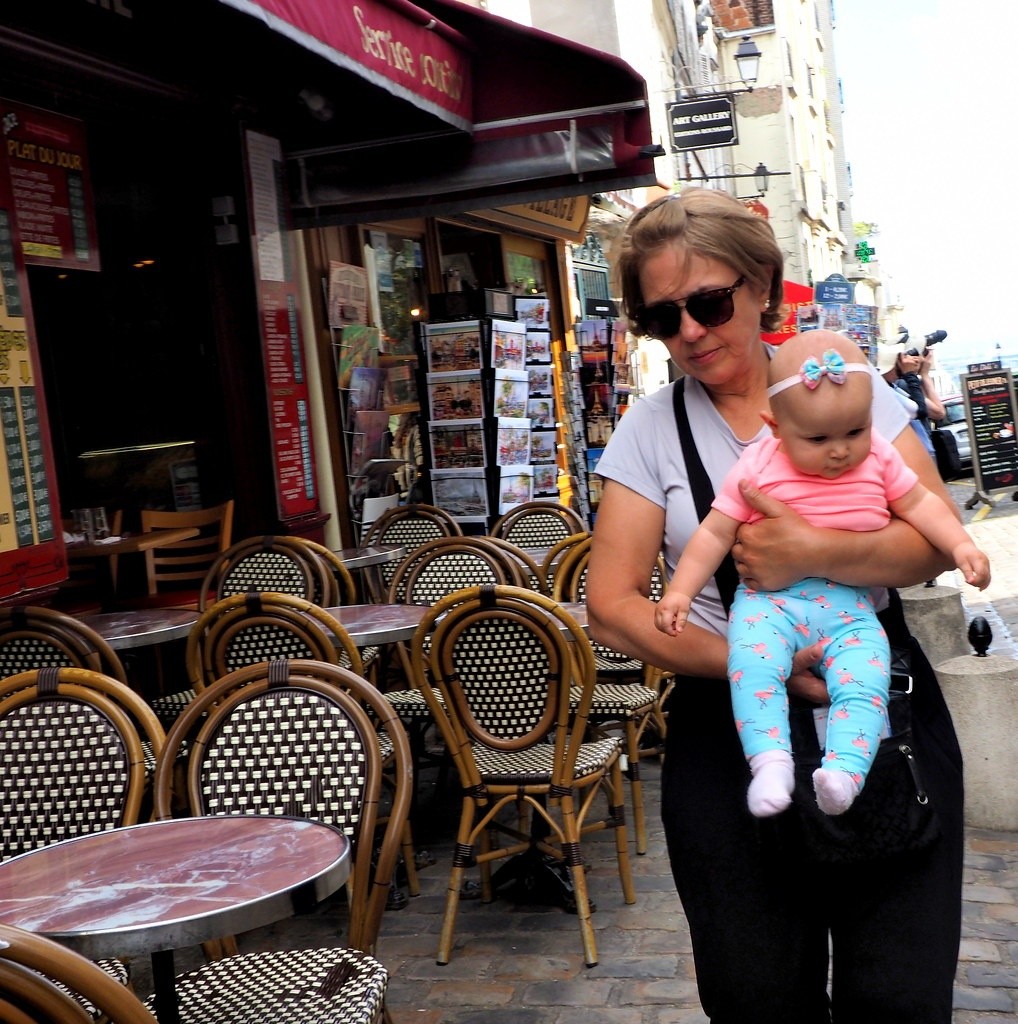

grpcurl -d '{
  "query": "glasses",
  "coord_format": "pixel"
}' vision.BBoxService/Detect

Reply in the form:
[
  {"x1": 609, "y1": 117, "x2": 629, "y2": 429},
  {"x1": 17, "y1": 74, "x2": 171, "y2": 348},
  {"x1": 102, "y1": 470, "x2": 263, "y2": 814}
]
[{"x1": 636, "y1": 275, "x2": 749, "y2": 341}]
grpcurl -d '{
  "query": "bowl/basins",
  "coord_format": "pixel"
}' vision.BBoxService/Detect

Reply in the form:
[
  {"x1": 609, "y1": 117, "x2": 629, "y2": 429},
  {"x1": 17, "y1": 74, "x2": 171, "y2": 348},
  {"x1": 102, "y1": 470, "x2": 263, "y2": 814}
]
[{"x1": 1000, "y1": 429, "x2": 1012, "y2": 436}]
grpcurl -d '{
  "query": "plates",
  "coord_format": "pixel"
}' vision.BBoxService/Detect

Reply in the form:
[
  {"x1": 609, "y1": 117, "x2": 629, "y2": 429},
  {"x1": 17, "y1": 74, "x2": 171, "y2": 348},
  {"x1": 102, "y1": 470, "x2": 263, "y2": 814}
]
[{"x1": 999, "y1": 434, "x2": 1014, "y2": 438}]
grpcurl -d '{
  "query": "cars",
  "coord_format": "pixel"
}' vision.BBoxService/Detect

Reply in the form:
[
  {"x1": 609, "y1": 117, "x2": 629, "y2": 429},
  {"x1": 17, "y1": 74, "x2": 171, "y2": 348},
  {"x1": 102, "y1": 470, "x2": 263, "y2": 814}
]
[{"x1": 934, "y1": 401, "x2": 973, "y2": 469}]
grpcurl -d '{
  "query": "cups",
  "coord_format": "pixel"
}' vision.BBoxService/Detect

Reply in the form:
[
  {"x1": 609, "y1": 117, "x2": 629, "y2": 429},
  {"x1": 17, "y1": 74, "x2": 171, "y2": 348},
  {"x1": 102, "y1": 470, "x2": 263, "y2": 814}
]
[{"x1": 1007, "y1": 423, "x2": 1014, "y2": 433}]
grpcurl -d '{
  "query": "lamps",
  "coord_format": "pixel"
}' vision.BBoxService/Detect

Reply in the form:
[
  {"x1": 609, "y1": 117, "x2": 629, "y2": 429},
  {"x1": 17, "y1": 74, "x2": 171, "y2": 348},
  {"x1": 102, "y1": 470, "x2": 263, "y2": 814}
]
[
  {"x1": 655, "y1": 35, "x2": 766, "y2": 101},
  {"x1": 736, "y1": 161, "x2": 770, "y2": 201}
]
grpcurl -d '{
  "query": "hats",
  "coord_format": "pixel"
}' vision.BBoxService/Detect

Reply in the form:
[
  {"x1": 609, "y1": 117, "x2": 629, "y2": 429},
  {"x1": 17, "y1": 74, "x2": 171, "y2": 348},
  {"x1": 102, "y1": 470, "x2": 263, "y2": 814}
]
[{"x1": 876, "y1": 341, "x2": 905, "y2": 375}]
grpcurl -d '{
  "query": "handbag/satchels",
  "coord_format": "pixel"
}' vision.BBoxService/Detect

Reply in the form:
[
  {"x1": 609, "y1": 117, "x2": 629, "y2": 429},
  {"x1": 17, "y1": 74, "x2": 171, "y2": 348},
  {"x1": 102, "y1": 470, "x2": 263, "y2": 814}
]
[
  {"x1": 930, "y1": 429, "x2": 962, "y2": 483},
  {"x1": 783, "y1": 698, "x2": 938, "y2": 864}
]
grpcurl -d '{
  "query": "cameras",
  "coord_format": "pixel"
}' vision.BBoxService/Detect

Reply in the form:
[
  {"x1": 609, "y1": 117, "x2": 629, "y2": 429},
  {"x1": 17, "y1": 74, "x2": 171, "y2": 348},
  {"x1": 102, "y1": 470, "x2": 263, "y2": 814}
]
[{"x1": 907, "y1": 330, "x2": 947, "y2": 357}]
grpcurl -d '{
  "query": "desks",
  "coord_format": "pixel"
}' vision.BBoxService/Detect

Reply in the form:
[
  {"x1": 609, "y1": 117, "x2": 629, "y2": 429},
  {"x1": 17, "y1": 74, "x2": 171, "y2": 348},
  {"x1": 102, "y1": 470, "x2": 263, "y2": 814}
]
[
  {"x1": 66, "y1": 525, "x2": 197, "y2": 597},
  {"x1": 458, "y1": 597, "x2": 597, "y2": 913},
  {"x1": 298, "y1": 542, "x2": 403, "y2": 601},
  {"x1": 0, "y1": 818, "x2": 351, "y2": 1024},
  {"x1": 299, "y1": 600, "x2": 436, "y2": 910},
  {"x1": 494, "y1": 546, "x2": 571, "y2": 592},
  {"x1": 73, "y1": 607, "x2": 198, "y2": 695}
]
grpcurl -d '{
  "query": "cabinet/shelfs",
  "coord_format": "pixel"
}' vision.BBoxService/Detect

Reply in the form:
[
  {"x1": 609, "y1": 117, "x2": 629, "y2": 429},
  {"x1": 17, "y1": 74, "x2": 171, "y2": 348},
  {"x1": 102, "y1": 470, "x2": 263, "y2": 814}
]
[{"x1": 330, "y1": 324, "x2": 404, "y2": 600}]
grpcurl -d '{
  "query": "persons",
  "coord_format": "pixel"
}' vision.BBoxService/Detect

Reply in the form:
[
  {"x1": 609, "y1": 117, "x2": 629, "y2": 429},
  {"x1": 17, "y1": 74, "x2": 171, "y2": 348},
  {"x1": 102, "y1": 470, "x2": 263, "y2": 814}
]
[
  {"x1": 586, "y1": 188, "x2": 964, "y2": 1024},
  {"x1": 878, "y1": 335, "x2": 947, "y2": 472},
  {"x1": 656, "y1": 330, "x2": 990, "y2": 814}
]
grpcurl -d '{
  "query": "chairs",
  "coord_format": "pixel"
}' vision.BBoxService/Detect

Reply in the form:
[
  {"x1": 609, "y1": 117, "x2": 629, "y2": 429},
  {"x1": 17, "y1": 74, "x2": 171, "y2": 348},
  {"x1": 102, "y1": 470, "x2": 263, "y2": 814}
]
[{"x1": 1, "y1": 499, "x2": 684, "y2": 1024}]
